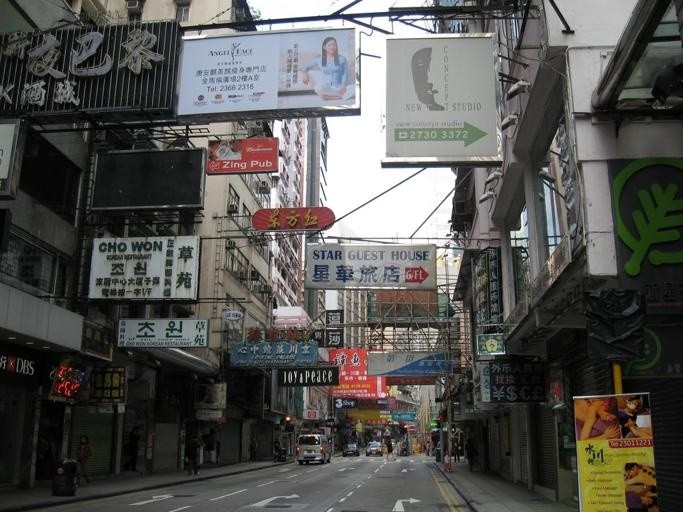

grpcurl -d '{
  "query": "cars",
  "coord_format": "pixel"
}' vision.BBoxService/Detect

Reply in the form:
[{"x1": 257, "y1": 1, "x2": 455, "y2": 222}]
[
  {"x1": 366, "y1": 441, "x2": 383, "y2": 456},
  {"x1": 341, "y1": 442, "x2": 359, "y2": 456}
]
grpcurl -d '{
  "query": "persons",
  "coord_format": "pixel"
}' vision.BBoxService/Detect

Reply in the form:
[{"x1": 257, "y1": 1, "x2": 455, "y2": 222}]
[
  {"x1": 302, "y1": 36, "x2": 348, "y2": 101},
  {"x1": 624, "y1": 462, "x2": 656, "y2": 487},
  {"x1": 620, "y1": 399, "x2": 645, "y2": 437},
  {"x1": 77, "y1": 434, "x2": 93, "y2": 485},
  {"x1": 124, "y1": 426, "x2": 140, "y2": 472},
  {"x1": 575, "y1": 396, "x2": 619, "y2": 439},
  {"x1": 383, "y1": 435, "x2": 463, "y2": 464},
  {"x1": 272, "y1": 436, "x2": 282, "y2": 464},
  {"x1": 624, "y1": 490, "x2": 656, "y2": 511},
  {"x1": 184, "y1": 437, "x2": 200, "y2": 475},
  {"x1": 248, "y1": 436, "x2": 257, "y2": 461}
]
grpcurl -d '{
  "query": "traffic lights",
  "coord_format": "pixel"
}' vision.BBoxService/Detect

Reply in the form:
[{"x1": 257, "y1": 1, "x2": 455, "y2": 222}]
[
  {"x1": 284, "y1": 415, "x2": 291, "y2": 431},
  {"x1": 433, "y1": 419, "x2": 439, "y2": 429},
  {"x1": 451, "y1": 424, "x2": 455, "y2": 433}
]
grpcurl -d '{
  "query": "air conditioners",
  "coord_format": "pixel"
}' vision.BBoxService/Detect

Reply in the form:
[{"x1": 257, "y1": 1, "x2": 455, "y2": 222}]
[
  {"x1": 224, "y1": 238, "x2": 235, "y2": 250},
  {"x1": 239, "y1": 269, "x2": 247, "y2": 279},
  {"x1": 260, "y1": 285, "x2": 270, "y2": 292},
  {"x1": 227, "y1": 203, "x2": 238, "y2": 213},
  {"x1": 258, "y1": 179, "x2": 269, "y2": 192},
  {"x1": 249, "y1": 271, "x2": 258, "y2": 280}
]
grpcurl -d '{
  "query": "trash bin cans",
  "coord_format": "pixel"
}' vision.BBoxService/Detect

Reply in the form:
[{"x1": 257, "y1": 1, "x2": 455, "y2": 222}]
[
  {"x1": 52, "y1": 462, "x2": 76, "y2": 496},
  {"x1": 278, "y1": 448, "x2": 286, "y2": 462},
  {"x1": 436, "y1": 449, "x2": 440, "y2": 462}
]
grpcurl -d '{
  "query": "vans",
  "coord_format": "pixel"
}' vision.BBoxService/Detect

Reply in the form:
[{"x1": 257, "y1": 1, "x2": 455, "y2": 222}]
[{"x1": 296, "y1": 434, "x2": 331, "y2": 466}]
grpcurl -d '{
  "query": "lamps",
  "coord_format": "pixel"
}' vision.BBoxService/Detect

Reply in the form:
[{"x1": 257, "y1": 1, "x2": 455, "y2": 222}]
[
  {"x1": 501, "y1": 113, "x2": 517, "y2": 134},
  {"x1": 478, "y1": 189, "x2": 497, "y2": 205},
  {"x1": 484, "y1": 168, "x2": 503, "y2": 184},
  {"x1": 505, "y1": 78, "x2": 530, "y2": 101}
]
